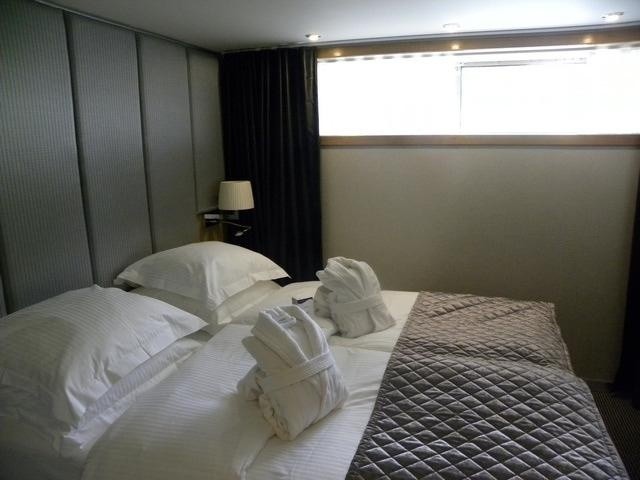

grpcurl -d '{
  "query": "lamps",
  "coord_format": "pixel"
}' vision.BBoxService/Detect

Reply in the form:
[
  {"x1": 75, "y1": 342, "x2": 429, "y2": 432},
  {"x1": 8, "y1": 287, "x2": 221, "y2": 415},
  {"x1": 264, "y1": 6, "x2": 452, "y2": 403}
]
[{"x1": 196, "y1": 178, "x2": 257, "y2": 238}]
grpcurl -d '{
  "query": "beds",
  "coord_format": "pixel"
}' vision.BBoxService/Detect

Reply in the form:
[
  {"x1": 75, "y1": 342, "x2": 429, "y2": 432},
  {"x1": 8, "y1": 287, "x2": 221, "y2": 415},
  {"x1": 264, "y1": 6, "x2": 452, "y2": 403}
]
[{"x1": 0, "y1": 283, "x2": 629, "y2": 480}]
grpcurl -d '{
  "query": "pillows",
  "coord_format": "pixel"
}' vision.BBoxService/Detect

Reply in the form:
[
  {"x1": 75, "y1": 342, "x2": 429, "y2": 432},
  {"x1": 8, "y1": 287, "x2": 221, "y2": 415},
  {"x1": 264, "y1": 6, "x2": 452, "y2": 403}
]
[
  {"x1": 0, "y1": 283, "x2": 209, "y2": 459},
  {"x1": 114, "y1": 240, "x2": 291, "y2": 335}
]
